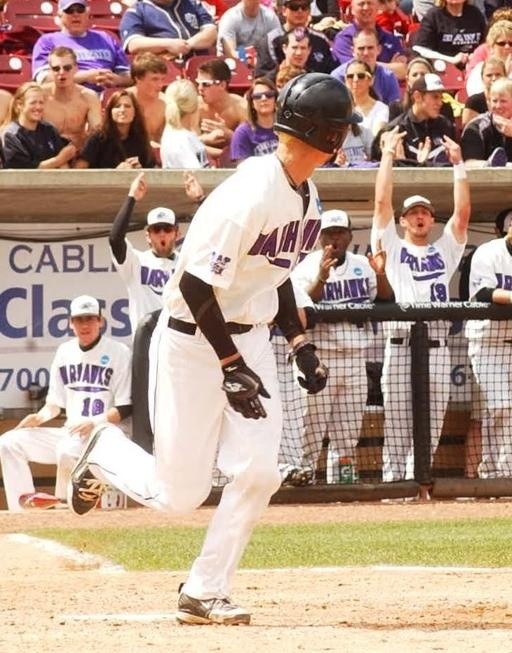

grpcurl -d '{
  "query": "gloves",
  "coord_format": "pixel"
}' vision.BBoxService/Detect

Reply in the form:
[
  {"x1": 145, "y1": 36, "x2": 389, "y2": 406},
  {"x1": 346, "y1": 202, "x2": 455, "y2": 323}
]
[
  {"x1": 222, "y1": 356, "x2": 272, "y2": 421},
  {"x1": 283, "y1": 338, "x2": 331, "y2": 394}
]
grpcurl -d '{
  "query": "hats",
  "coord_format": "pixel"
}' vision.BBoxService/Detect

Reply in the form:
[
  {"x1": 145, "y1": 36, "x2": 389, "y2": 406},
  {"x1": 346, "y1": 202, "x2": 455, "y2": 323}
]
[
  {"x1": 70, "y1": 293, "x2": 101, "y2": 321},
  {"x1": 57, "y1": 0, "x2": 88, "y2": 14},
  {"x1": 402, "y1": 194, "x2": 435, "y2": 218},
  {"x1": 147, "y1": 206, "x2": 176, "y2": 228},
  {"x1": 319, "y1": 208, "x2": 352, "y2": 230},
  {"x1": 412, "y1": 71, "x2": 447, "y2": 93}
]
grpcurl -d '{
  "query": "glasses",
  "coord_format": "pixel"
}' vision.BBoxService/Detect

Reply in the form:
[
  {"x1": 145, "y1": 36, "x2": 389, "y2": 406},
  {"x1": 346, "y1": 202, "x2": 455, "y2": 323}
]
[
  {"x1": 195, "y1": 78, "x2": 226, "y2": 88},
  {"x1": 63, "y1": 5, "x2": 87, "y2": 15},
  {"x1": 345, "y1": 71, "x2": 373, "y2": 81},
  {"x1": 151, "y1": 224, "x2": 175, "y2": 234},
  {"x1": 250, "y1": 90, "x2": 277, "y2": 100},
  {"x1": 285, "y1": 3, "x2": 309, "y2": 12},
  {"x1": 50, "y1": 64, "x2": 73, "y2": 72},
  {"x1": 493, "y1": 42, "x2": 512, "y2": 47}
]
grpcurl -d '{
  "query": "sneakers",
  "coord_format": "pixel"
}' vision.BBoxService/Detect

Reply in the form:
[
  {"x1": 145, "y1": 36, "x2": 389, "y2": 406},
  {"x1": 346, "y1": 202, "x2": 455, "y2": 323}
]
[
  {"x1": 178, "y1": 587, "x2": 252, "y2": 629},
  {"x1": 68, "y1": 420, "x2": 118, "y2": 517},
  {"x1": 486, "y1": 147, "x2": 507, "y2": 167}
]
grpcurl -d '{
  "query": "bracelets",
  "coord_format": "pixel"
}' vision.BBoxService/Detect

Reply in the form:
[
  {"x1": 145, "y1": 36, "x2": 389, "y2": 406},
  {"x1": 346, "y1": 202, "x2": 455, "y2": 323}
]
[
  {"x1": 453, "y1": 164, "x2": 468, "y2": 180},
  {"x1": 92, "y1": 413, "x2": 107, "y2": 427},
  {"x1": 376, "y1": 271, "x2": 386, "y2": 275},
  {"x1": 317, "y1": 275, "x2": 327, "y2": 284}
]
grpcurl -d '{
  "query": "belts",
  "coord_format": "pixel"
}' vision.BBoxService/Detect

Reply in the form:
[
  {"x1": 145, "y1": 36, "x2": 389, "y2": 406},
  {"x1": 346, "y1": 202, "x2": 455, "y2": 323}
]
[
  {"x1": 168, "y1": 315, "x2": 254, "y2": 336},
  {"x1": 388, "y1": 337, "x2": 449, "y2": 349}
]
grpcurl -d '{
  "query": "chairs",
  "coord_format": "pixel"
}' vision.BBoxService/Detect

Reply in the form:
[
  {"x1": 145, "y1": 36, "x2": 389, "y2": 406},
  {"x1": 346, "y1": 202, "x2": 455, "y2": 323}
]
[{"x1": 0, "y1": 0, "x2": 466, "y2": 167}]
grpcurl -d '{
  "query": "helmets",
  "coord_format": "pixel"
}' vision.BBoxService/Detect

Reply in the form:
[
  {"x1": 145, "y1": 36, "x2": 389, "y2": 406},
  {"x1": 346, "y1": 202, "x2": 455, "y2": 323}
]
[{"x1": 273, "y1": 70, "x2": 363, "y2": 154}]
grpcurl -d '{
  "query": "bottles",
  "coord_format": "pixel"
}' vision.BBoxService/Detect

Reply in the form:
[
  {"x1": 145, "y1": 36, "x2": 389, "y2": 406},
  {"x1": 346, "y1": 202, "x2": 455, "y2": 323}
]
[
  {"x1": 337, "y1": 456, "x2": 354, "y2": 483},
  {"x1": 19, "y1": 491, "x2": 69, "y2": 509}
]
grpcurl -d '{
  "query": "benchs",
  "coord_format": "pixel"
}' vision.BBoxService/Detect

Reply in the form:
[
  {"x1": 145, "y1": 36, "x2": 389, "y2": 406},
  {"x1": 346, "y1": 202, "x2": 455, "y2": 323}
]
[{"x1": 0, "y1": 408, "x2": 470, "y2": 508}]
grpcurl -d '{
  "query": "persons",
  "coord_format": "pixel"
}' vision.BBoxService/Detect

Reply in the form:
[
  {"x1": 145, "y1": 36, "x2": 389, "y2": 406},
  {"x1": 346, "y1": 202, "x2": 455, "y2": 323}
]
[
  {"x1": 0, "y1": 295, "x2": 133, "y2": 514},
  {"x1": 108, "y1": 172, "x2": 205, "y2": 344},
  {"x1": 464, "y1": 217, "x2": 512, "y2": 478},
  {"x1": 370, "y1": 126, "x2": 470, "y2": 503},
  {"x1": 1, "y1": 0, "x2": 512, "y2": 167},
  {"x1": 289, "y1": 209, "x2": 377, "y2": 486},
  {"x1": 69, "y1": 71, "x2": 352, "y2": 629}
]
